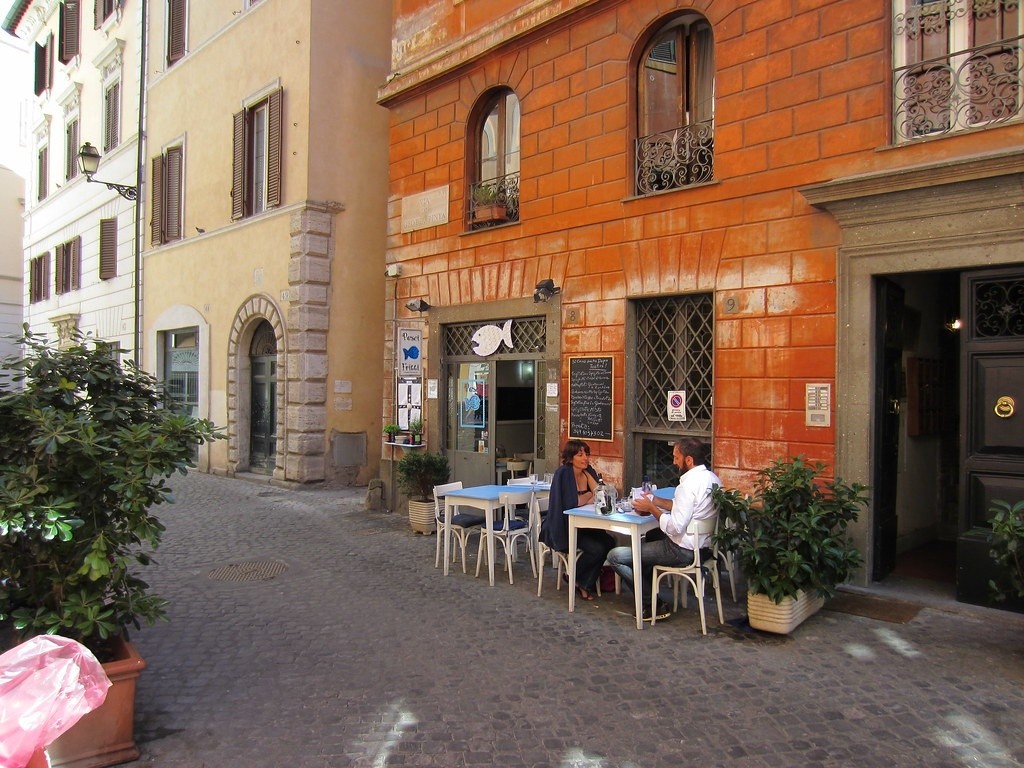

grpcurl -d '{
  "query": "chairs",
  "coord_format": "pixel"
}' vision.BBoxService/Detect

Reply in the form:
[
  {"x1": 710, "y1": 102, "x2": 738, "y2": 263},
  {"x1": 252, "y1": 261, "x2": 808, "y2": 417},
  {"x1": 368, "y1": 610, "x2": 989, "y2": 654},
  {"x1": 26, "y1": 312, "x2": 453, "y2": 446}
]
[
  {"x1": 495, "y1": 452, "x2": 535, "y2": 486},
  {"x1": 432, "y1": 480, "x2": 488, "y2": 574},
  {"x1": 534, "y1": 497, "x2": 602, "y2": 598},
  {"x1": 681, "y1": 493, "x2": 756, "y2": 609},
  {"x1": 650, "y1": 518, "x2": 724, "y2": 636},
  {"x1": 475, "y1": 486, "x2": 537, "y2": 585}
]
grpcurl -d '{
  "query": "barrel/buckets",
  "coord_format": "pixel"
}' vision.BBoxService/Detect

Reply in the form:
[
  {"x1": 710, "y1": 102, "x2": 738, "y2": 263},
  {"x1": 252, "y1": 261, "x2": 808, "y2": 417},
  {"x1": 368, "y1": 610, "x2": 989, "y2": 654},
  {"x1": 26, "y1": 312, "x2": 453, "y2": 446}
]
[{"x1": 395, "y1": 435, "x2": 407, "y2": 444}]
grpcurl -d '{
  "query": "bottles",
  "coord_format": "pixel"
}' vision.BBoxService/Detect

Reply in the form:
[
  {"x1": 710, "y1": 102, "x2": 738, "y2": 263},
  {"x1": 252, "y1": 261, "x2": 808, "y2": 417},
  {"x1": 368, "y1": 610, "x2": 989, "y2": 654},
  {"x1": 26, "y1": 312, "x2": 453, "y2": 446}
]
[
  {"x1": 598, "y1": 472, "x2": 612, "y2": 514},
  {"x1": 641, "y1": 476, "x2": 652, "y2": 516}
]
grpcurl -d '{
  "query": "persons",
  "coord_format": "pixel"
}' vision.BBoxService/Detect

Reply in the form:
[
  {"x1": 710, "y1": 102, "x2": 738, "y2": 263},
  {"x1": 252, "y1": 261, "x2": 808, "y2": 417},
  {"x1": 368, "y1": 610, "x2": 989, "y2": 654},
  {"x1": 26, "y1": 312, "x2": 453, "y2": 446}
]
[
  {"x1": 606, "y1": 437, "x2": 727, "y2": 621},
  {"x1": 537, "y1": 438, "x2": 616, "y2": 601}
]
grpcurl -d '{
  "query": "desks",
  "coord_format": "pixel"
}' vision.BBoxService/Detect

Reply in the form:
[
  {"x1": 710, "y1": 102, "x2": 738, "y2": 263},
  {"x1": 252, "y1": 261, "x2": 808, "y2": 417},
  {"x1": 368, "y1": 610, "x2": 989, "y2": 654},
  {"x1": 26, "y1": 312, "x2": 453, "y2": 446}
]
[
  {"x1": 440, "y1": 484, "x2": 542, "y2": 586},
  {"x1": 563, "y1": 486, "x2": 676, "y2": 630}
]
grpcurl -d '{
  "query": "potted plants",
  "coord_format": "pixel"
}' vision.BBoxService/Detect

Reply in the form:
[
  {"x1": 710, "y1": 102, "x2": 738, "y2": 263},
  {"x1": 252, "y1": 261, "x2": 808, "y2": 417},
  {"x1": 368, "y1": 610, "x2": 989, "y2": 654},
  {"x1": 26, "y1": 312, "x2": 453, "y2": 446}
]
[
  {"x1": 395, "y1": 449, "x2": 452, "y2": 535},
  {"x1": 0, "y1": 323, "x2": 232, "y2": 767},
  {"x1": 383, "y1": 424, "x2": 402, "y2": 442},
  {"x1": 469, "y1": 184, "x2": 507, "y2": 219},
  {"x1": 705, "y1": 453, "x2": 873, "y2": 635},
  {"x1": 409, "y1": 418, "x2": 424, "y2": 445}
]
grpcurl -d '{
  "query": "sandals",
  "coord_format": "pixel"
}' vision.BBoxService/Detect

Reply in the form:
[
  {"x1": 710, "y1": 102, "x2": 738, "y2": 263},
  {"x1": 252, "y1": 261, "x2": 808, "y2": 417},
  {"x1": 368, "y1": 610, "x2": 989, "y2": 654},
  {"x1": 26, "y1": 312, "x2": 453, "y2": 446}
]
[{"x1": 575, "y1": 585, "x2": 594, "y2": 601}]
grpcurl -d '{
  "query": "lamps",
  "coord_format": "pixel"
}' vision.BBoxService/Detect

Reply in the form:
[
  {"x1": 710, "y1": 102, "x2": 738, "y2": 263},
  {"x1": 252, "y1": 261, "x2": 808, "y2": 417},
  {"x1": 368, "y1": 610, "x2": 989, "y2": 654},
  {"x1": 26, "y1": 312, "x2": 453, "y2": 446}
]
[{"x1": 75, "y1": 141, "x2": 138, "y2": 201}]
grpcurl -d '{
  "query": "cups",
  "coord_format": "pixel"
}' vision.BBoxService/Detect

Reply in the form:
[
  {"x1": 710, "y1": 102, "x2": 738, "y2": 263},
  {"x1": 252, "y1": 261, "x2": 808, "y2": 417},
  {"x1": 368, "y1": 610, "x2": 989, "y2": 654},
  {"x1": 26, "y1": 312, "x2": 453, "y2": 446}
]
[
  {"x1": 622, "y1": 497, "x2": 632, "y2": 512},
  {"x1": 530, "y1": 474, "x2": 538, "y2": 485},
  {"x1": 543, "y1": 472, "x2": 553, "y2": 486}
]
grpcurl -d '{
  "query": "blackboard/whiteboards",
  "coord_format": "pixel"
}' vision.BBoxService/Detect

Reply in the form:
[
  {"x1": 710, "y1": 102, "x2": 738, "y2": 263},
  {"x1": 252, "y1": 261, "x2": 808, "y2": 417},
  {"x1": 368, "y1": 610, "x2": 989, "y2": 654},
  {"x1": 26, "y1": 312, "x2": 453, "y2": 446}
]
[{"x1": 567, "y1": 356, "x2": 615, "y2": 442}]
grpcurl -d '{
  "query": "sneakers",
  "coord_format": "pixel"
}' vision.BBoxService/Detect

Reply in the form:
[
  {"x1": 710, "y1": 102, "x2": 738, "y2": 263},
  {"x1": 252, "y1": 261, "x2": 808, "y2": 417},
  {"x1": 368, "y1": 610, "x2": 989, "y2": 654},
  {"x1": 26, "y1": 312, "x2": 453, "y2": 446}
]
[{"x1": 632, "y1": 602, "x2": 671, "y2": 621}]
